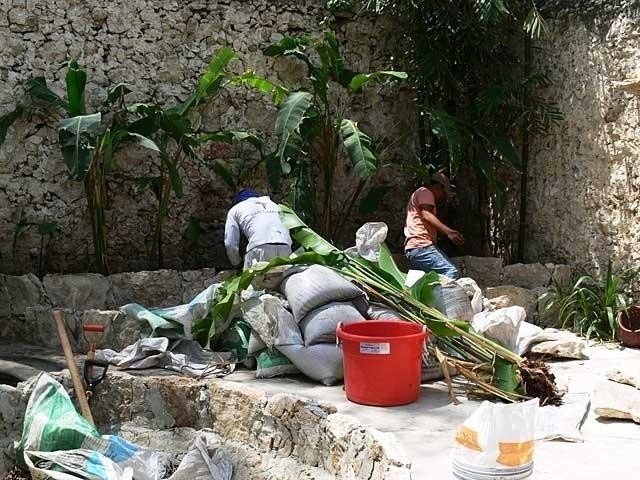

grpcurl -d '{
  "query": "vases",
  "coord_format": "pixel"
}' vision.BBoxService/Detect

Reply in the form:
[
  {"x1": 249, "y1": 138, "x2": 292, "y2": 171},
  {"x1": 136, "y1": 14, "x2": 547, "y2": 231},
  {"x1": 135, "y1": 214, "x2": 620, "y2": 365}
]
[{"x1": 616, "y1": 305, "x2": 640, "y2": 348}]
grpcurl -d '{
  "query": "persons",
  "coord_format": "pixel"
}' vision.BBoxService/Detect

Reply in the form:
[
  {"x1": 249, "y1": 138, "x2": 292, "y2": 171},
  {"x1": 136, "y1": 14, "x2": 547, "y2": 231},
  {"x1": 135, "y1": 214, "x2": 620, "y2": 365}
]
[
  {"x1": 402, "y1": 172, "x2": 467, "y2": 280},
  {"x1": 222, "y1": 188, "x2": 294, "y2": 275}
]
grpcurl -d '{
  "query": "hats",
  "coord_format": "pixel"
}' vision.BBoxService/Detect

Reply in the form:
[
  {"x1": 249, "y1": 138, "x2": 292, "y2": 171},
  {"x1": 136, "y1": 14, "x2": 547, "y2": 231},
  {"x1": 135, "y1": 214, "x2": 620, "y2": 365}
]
[{"x1": 431, "y1": 173, "x2": 451, "y2": 202}]
